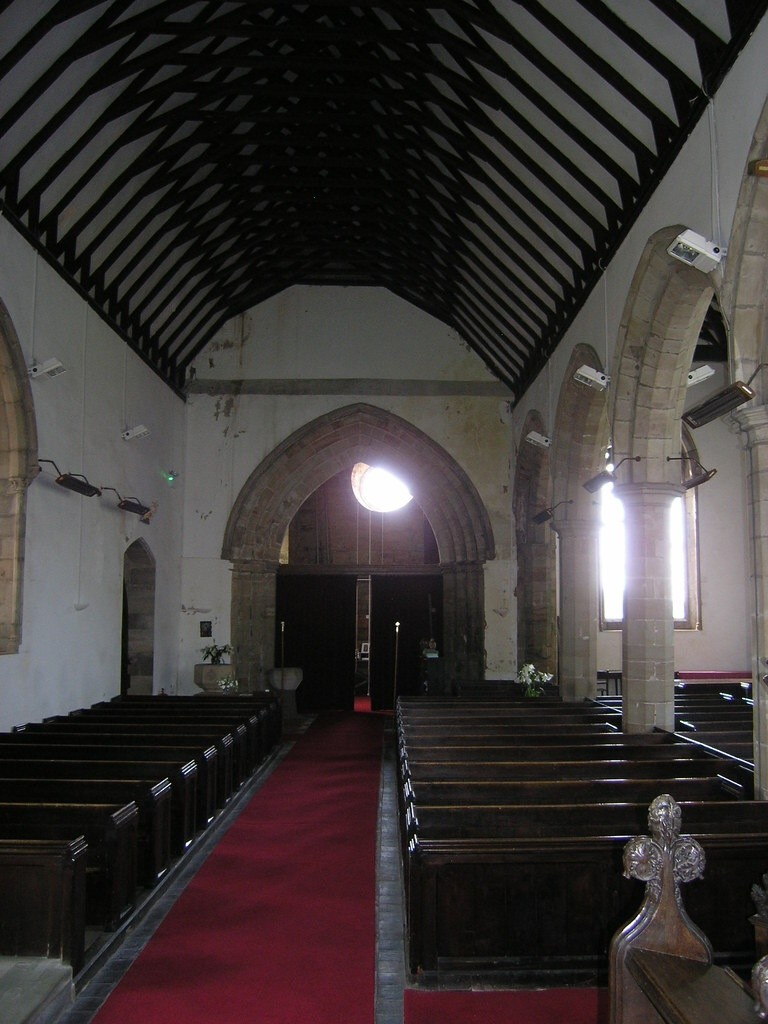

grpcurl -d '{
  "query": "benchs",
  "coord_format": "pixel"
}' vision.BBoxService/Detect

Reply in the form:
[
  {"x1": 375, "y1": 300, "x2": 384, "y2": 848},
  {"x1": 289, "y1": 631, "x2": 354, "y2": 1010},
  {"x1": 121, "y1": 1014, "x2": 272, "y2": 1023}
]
[
  {"x1": 0, "y1": 689, "x2": 283, "y2": 973},
  {"x1": 395, "y1": 671, "x2": 768, "y2": 983}
]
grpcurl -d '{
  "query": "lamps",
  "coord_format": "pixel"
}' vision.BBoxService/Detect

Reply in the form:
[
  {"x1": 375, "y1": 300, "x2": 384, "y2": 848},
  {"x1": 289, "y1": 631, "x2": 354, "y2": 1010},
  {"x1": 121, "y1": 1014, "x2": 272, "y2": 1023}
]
[
  {"x1": 102, "y1": 486, "x2": 150, "y2": 516},
  {"x1": 573, "y1": 365, "x2": 611, "y2": 391},
  {"x1": 525, "y1": 431, "x2": 553, "y2": 449},
  {"x1": 667, "y1": 229, "x2": 728, "y2": 274},
  {"x1": 36, "y1": 459, "x2": 101, "y2": 497},
  {"x1": 28, "y1": 358, "x2": 68, "y2": 384},
  {"x1": 583, "y1": 456, "x2": 642, "y2": 493},
  {"x1": 121, "y1": 425, "x2": 150, "y2": 442},
  {"x1": 680, "y1": 361, "x2": 768, "y2": 429},
  {"x1": 533, "y1": 499, "x2": 573, "y2": 526},
  {"x1": 668, "y1": 457, "x2": 717, "y2": 488}
]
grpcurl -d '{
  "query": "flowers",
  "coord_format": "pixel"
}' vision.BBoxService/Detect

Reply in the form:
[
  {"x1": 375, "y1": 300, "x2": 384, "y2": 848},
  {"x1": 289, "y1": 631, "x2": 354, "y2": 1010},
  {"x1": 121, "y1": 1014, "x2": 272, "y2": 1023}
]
[
  {"x1": 201, "y1": 643, "x2": 235, "y2": 664},
  {"x1": 516, "y1": 663, "x2": 554, "y2": 699},
  {"x1": 217, "y1": 677, "x2": 239, "y2": 696}
]
